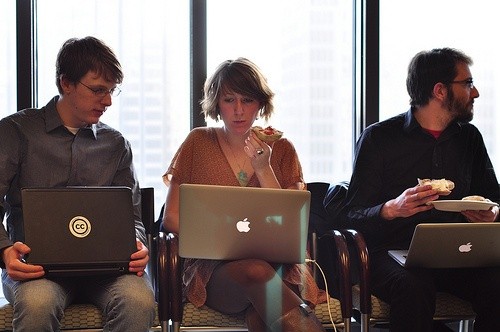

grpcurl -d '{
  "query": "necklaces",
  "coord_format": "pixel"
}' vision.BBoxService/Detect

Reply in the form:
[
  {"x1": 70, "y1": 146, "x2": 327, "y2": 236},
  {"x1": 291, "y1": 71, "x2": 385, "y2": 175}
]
[{"x1": 226, "y1": 135, "x2": 248, "y2": 182}]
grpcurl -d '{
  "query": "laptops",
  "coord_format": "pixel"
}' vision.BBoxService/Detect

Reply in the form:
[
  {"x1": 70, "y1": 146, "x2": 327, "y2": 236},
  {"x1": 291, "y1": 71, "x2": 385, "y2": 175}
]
[
  {"x1": 180, "y1": 183, "x2": 309, "y2": 265},
  {"x1": 388, "y1": 222, "x2": 500, "y2": 268},
  {"x1": 22, "y1": 186, "x2": 135, "y2": 275}
]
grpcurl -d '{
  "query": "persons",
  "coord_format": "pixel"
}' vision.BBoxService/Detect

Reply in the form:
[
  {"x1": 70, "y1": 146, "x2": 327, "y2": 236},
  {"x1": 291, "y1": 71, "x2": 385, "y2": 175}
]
[
  {"x1": 329, "y1": 48, "x2": 500, "y2": 332},
  {"x1": 0, "y1": 35, "x2": 155, "y2": 332},
  {"x1": 162, "y1": 57, "x2": 329, "y2": 332}
]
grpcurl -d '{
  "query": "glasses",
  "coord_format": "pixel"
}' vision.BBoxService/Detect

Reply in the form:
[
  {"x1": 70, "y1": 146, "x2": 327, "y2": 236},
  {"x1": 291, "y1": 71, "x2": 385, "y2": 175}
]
[
  {"x1": 443, "y1": 79, "x2": 474, "y2": 90},
  {"x1": 77, "y1": 81, "x2": 122, "y2": 97}
]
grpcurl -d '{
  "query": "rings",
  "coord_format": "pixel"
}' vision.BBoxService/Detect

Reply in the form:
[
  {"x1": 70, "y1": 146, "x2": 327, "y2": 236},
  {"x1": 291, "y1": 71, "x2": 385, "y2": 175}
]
[{"x1": 256, "y1": 149, "x2": 264, "y2": 154}]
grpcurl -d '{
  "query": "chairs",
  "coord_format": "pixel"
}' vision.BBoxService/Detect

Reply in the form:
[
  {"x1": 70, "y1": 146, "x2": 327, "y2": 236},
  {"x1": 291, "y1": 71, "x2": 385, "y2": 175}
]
[{"x1": 0, "y1": 182, "x2": 476, "y2": 332}]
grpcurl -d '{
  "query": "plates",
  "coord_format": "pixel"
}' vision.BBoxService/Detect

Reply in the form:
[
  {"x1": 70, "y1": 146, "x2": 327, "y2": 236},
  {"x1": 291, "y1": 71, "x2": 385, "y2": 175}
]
[{"x1": 430, "y1": 200, "x2": 499, "y2": 212}]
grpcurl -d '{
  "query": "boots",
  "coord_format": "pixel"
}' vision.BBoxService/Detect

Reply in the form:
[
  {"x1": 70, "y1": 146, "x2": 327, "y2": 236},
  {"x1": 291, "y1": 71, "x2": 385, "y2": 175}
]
[{"x1": 270, "y1": 304, "x2": 326, "y2": 332}]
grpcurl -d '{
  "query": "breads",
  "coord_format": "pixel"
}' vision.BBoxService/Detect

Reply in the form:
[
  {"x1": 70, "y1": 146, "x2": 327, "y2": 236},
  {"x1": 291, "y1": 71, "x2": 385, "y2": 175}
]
[
  {"x1": 422, "y1": 178, "x2": 455, "y2": 196},
  {"x1": 253, "y1": 125, "x2": 283, "y2": 142}
]
